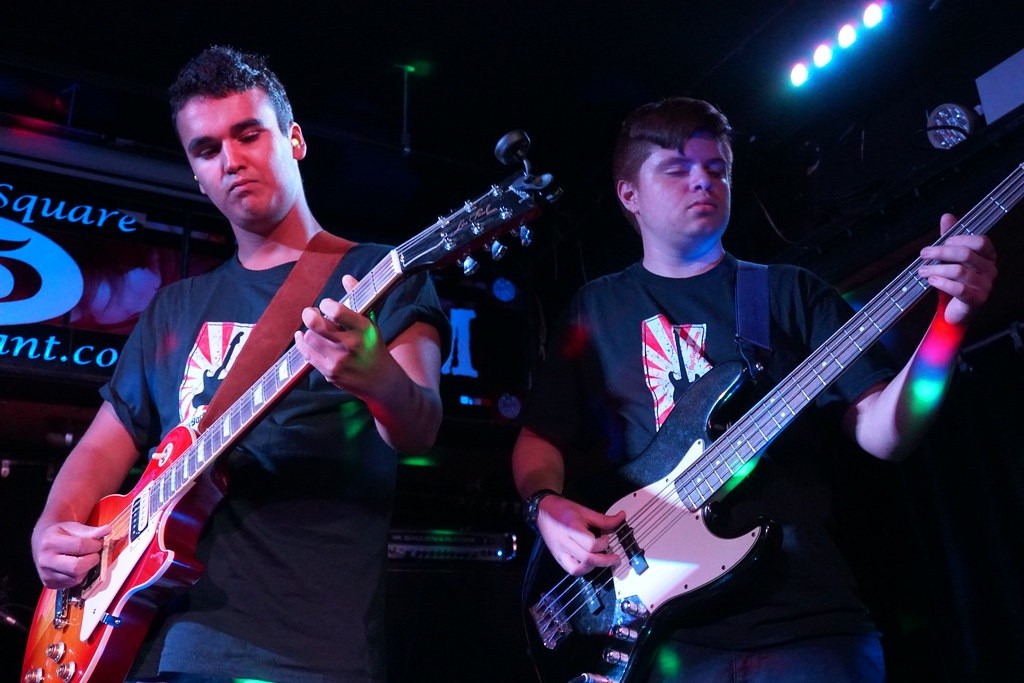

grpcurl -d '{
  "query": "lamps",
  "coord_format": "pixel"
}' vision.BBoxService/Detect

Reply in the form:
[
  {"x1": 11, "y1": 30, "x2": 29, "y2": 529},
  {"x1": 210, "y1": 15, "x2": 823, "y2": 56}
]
[{"x1": 926, "y1": 49, "x2": 1024, "y2": 150}]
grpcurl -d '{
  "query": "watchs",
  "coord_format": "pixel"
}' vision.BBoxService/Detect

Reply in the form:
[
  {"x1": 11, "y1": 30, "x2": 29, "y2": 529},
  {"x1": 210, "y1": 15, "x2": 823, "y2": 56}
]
[{"x1": 522, "y1": 489, "x2": 561, "y2": 535}]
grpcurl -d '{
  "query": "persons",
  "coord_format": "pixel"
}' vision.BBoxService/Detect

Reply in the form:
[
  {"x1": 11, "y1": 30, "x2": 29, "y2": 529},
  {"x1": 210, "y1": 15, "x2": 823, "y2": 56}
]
[
  {"x1": 30, "y1": 44, "x2": 453, "y2": 683},
  {"x1": 512, "y1": 97, "x2": 999, "y2": 683}
]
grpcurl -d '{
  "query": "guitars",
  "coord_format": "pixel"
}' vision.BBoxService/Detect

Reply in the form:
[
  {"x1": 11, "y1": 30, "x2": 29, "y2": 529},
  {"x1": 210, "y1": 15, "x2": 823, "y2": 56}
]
[
  {"x1": 18, "y1": 129, "x2": 564, "y2": 683},
  {"x1": 517, "y1": 156, "x2": 1024, "y2": 683}
]
